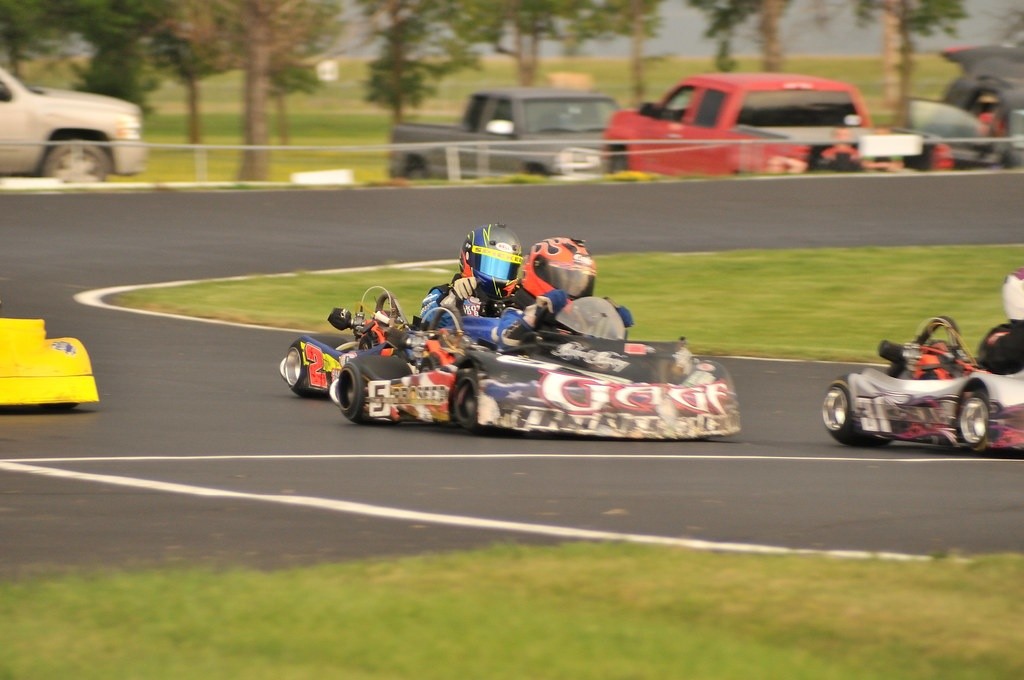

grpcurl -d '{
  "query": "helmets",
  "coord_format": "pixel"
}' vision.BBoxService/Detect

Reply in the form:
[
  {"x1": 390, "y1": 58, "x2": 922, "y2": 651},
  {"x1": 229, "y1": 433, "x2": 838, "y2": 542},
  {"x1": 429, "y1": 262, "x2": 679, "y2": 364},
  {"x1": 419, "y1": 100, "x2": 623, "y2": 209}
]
[
  {"x1": 1003, "y1": 268, "x2": 1024, "y2": 320},
  {"x1": 460, "y1": 223, "x2": 521, "y2": 299},
  {"x1": 518, "y1": 237, "x2": 596, "y2": 310}
]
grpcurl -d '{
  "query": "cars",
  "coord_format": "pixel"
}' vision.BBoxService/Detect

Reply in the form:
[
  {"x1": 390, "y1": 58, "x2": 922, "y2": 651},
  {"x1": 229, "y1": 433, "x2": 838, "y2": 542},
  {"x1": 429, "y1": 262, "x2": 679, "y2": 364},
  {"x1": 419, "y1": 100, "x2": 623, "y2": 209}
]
[
  {"x1": 882, "y1": 92, "x2": 1003, "y2": 172},
  {"x1": 594, "y1": 70, "x2": 911, "y2": 174},
  {"x1": 0, "y1": 68, "x2": 143, "y2": 182}
]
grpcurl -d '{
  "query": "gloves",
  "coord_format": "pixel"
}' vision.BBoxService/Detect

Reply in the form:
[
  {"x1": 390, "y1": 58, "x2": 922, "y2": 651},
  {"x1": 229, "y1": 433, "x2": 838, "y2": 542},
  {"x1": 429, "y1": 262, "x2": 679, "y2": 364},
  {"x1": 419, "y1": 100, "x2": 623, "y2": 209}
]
[
  {"x1": 617, "y1": 305, "x2": 634, "y2": 328},
  {"x1": 453, "y1": 276, "x2": 477, "y2": 300},
  {"x1": 525, "y1": 289, "x2": 566, "y2": 319}
]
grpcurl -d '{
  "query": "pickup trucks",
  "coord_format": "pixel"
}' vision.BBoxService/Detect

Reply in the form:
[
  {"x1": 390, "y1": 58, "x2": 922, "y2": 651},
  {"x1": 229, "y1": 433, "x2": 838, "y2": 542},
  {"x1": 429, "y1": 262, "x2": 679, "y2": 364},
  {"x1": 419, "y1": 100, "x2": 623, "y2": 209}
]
[{"x1": 379, "y1": 84, "x2": 621, "y2": 183}]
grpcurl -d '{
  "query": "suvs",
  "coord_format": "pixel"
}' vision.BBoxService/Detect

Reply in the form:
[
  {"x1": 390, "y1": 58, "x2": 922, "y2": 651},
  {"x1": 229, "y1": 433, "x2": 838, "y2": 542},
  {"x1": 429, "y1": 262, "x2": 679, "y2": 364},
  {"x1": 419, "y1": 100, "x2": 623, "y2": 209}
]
[{"x1": 941, "y1": 44, "x2": 1024, "y2": 172}]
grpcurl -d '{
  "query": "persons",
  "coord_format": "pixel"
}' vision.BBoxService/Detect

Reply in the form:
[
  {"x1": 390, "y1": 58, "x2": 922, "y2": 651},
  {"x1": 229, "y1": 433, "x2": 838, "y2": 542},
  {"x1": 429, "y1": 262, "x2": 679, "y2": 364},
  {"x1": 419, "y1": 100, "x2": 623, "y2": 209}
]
[
  {"x1": 978, "y1": 267, "x2": 1024, "y2": 375},
  {"x1": 420, "y1": 223, "x2": 522, "y2": 329},
  {"x1": 863, "y1": 124, "x2": 931, "y2": 174},
  {"x1": 817, "y1": 128, "x2": 860, "y2": 173},
  {"x1": 497, "y1": 237, "x2": 634, "y2": 351}
]
